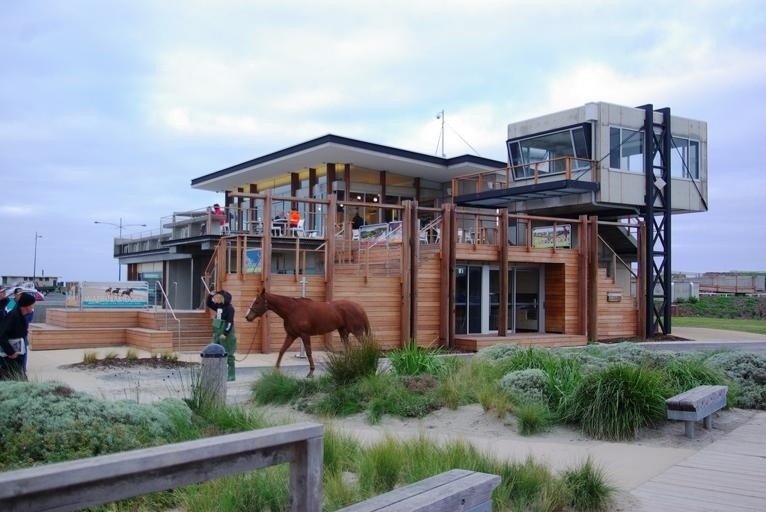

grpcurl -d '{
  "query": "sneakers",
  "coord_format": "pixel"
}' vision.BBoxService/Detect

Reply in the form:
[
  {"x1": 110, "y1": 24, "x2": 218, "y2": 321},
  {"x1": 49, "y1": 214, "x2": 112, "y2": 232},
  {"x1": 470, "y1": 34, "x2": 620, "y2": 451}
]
[{"x1": 228, "y1": 378, "x2": 235, "y2": 381}]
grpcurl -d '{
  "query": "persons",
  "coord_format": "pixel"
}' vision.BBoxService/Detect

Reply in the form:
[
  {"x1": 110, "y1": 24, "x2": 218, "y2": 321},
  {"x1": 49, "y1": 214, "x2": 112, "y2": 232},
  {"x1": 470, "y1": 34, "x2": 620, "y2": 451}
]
[
  {"x1": 0, "y1": 285, "x2": 35, "y2": 382},
  {"x1": 271, "y1": 209, "x2": 288, "y2": 236},
  {"x1": 288, "y1": 208, "x2": 301, "y2": 237},
  {"x1": 199, "y1": 204, "x2": 234, "y2": 236},
  {"x1": 206, "y1": 289, "x2": 236, "y2": 381}
]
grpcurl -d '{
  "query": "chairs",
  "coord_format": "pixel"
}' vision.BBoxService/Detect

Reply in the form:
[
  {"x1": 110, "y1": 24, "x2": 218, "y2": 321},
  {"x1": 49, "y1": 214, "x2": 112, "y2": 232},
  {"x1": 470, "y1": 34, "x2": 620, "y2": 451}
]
[{"x1": 258, "y1": 217, "x2": 307, "y2": 237}]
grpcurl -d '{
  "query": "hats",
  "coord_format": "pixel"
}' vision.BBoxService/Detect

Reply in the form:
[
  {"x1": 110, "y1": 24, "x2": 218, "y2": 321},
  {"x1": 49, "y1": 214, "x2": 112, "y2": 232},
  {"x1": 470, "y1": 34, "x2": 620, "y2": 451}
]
[{"x1": 18, "y1": 293, "x2": 35, "y2": 306}]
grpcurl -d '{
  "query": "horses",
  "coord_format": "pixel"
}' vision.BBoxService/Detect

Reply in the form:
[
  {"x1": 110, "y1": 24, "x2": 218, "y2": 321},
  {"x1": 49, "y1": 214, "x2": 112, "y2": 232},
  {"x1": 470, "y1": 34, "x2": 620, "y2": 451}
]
[{"x1": 245, "y1": 288, "x2": 372, "y2": 379}]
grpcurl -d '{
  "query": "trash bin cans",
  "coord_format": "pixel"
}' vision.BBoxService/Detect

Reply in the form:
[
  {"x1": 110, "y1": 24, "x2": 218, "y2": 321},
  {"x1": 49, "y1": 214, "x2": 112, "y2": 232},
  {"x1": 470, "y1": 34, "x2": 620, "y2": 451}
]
[{"x1": 200, "y1": 343, "x2": 228, "y2": 409}]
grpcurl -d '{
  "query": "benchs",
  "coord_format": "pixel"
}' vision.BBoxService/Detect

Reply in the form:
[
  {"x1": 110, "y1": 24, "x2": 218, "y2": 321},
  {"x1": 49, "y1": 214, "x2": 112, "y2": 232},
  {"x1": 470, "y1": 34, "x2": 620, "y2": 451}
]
[
  {"x1": 0, "y1": 422, "x2": 503, "y2": 512},
  {"x1": 664, "y1": 384, "x2": 727, "y2": 437}
]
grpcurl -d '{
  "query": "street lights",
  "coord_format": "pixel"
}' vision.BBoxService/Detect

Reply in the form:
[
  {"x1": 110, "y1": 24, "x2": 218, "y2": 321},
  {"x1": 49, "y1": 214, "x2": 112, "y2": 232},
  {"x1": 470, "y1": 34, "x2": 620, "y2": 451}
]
[
  {"x1": 33, "y1": 231, "x2": 43, "y2": 281},
  {"x1": 92, "y1": 214, "x2": 148, "y2": 282}
]
